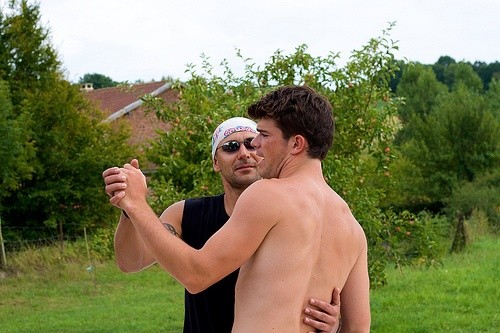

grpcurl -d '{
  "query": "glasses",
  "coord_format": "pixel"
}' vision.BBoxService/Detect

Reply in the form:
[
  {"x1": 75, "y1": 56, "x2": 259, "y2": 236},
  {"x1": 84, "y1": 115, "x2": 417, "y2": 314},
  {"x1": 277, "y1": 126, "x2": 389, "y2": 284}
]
[{"x1": 216, "y1": 138, "x2": 256, "y2": 152}]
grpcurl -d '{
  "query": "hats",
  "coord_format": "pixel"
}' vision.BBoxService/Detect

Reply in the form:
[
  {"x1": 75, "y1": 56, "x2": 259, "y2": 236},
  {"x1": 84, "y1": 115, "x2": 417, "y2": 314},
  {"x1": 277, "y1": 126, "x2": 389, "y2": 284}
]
[{"x1": 212, "y1": 117, "x2": 261, "y2": 159}]
